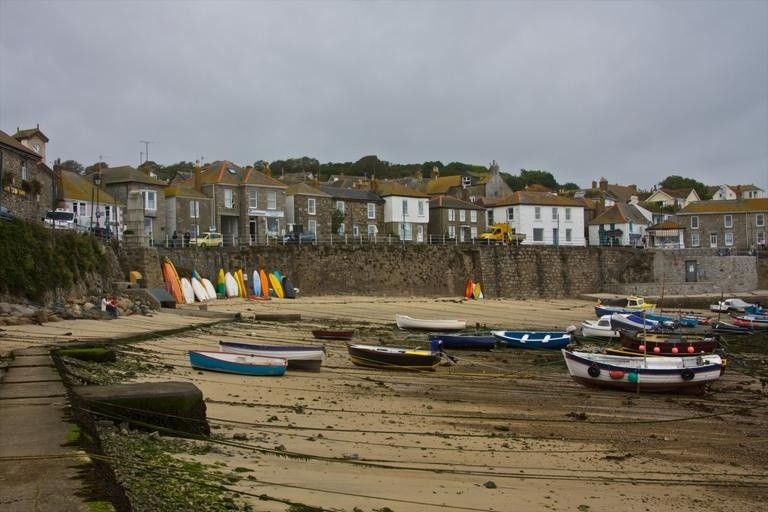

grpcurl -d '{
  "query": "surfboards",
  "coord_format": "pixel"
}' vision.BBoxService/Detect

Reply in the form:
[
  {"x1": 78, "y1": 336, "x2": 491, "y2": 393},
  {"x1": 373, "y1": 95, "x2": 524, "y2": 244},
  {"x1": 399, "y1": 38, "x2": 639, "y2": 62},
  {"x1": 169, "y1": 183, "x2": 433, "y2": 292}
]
[
  {"x1": 466, "y1": 278, "x2": 483, "y2": 300},
  {"x1": 162, "y1": 255, "x2": 295, "y2": 304}
]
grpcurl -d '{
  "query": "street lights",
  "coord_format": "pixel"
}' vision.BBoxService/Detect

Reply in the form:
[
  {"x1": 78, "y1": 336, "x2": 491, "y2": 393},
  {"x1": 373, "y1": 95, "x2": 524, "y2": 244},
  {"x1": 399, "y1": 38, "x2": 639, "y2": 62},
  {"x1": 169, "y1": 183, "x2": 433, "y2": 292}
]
[{"x1": 92, "y1": 169, "x2": 104, "y2": 228}]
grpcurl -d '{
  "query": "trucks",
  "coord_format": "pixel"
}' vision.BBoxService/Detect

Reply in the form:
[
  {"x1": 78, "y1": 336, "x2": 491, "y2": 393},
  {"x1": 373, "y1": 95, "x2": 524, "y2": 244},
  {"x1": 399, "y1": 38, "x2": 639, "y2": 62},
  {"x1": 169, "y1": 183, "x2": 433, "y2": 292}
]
[{"x1": 480, "y1": 222, "x2": 527, "y2": 245}]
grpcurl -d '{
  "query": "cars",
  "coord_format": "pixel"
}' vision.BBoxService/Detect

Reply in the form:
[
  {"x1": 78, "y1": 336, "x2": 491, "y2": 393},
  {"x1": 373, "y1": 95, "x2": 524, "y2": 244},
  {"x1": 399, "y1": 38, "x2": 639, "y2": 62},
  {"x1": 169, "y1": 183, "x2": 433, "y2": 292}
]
[
  {"x1": 188, "y1": 231, "x2": 224, "y2": 248},
  {"x1": 42, "y1": 207, "x2": 79, "y2": 231},
  {"x1": 278, "y1": 229, "x2": 316, "y2": 246}
]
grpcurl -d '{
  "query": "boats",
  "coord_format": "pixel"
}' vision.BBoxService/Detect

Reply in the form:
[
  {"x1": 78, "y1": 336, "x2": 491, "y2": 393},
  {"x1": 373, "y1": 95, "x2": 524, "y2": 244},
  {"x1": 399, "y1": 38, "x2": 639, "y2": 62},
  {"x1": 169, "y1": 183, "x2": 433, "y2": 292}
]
[
  {"x1": 187, "y1": 349, "x2": 289, "y2": 376},
  {"x1": 310, "y1": 327, "x2": 355, "y2": 342},
  {"x1": 427, "y1": 333, "x2": 497, "y2": 351},
  {"x1": 395, "y1": 312, "x2": 467, "y2": 331},
  {"x1": 217, "y1": 340, "x2": 326, "y2": 374},
  {"x1": 160, "y1": 255, "x2": 296, "y2": 305},
  {"x1": 560, "y1": 347, "x2": 727, "y2": 392},
  {"x1": 489, "y1": 325, "x2": 577, "y2": 348},
  {"x1": 580, "y1": 295, "x2": 768, "y2": 350},
  {"x1": 346, "y1": 343, "x2": 442, "y2": 373},
  {"x1": 463, "y1": 277, "x2": 487, "y2": 303}
]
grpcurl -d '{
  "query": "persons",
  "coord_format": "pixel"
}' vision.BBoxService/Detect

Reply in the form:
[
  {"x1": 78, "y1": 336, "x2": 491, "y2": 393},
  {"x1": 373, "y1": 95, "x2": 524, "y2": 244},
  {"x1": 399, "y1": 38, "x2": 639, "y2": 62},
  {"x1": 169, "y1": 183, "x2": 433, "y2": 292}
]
[
  {"x1": 642, "y1": 235, "x2": 647, "y2": 248},
  {"x1": 747, "y1": 242, "x2": 756, "y2": 256},
  {"x1": 607, "y1": 236, "x2": 619, "y2": 245},
  {"x1": 171, "y1": 227, "x2": 191, "y2": 247},
  {"x1": 101, "y1": 294, "x2": 117, "y2": 318}
]
